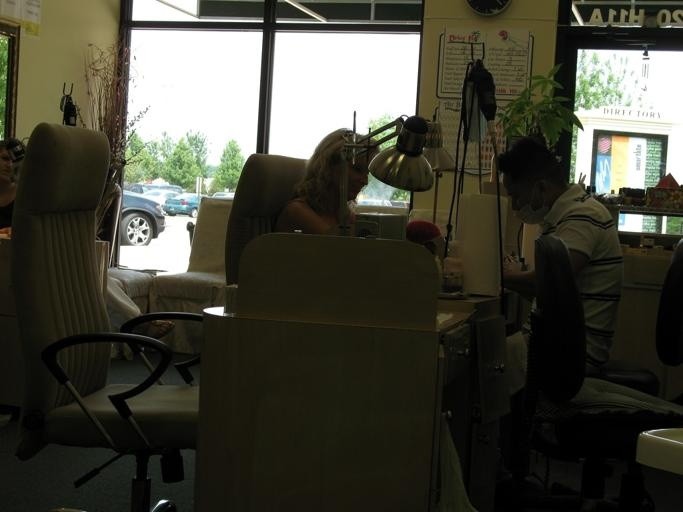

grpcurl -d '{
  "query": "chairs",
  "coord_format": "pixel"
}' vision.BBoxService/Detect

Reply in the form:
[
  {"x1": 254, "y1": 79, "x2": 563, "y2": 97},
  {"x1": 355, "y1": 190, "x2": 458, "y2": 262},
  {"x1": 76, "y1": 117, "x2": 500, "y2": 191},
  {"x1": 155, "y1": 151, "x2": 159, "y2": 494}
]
[
  {"x1": 146, "y1": 197, "x2": 234, "y2": 357},
  {"x1": 10, "y1": 123, "x2": 203, "y2": 511},
  {"x1": 225, "y1": 153, "x2": 310, "y2": 286},
  {"x1": 107, "y1": 265, "x2": 153, "y2": 361},
  {"x1": 511, "y1": 235, "x2": 683, "y2": 512},
  {"x1": 614, "y1": 237, "x2": 683, "y2": 406}
]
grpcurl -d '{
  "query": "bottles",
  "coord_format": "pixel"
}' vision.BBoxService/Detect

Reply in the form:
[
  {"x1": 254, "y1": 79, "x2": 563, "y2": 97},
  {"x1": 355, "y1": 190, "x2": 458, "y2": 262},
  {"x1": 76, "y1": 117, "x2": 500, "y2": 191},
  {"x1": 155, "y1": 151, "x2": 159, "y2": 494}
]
[{"x1": 441, "y1": 240, "x2": 466, "y2": 294}]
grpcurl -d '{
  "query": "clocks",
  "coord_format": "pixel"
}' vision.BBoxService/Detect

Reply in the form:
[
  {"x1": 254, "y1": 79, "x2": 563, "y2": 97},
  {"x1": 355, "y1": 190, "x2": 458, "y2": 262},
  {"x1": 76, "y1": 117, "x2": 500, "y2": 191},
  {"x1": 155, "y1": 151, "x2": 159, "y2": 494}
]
[{"x1": 465, "y1": 0, "x2": 511, "y2": 17}]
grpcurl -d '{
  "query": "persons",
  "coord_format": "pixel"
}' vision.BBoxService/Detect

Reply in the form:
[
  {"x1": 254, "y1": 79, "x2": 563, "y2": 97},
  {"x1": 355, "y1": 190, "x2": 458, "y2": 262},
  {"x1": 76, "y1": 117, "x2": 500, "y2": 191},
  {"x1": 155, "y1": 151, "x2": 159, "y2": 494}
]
[
  {"x1": 494, "y1": 135, "x2": 624, "y2": 374},
  {"x1": 274, "y1": 128, "x2": 379, "y2": 235},
  {"x1": 0, "y1": 140, "x2": 16, "y2": 230}
]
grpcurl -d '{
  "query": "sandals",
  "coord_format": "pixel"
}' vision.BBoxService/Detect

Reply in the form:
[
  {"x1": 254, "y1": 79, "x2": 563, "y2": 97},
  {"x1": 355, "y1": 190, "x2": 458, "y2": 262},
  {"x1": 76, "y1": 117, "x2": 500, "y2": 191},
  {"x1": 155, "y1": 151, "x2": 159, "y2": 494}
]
[{"x1": 133, "y1": 319, "x2": 175, "y2": 344}]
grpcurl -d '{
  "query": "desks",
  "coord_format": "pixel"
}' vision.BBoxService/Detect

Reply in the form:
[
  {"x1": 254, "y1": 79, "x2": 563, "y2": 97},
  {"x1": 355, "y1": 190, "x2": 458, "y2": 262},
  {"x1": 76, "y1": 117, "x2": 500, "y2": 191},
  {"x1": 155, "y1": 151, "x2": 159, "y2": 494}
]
[{"x1": 193, "y1": 294, "x2": 501, "y2": 511}]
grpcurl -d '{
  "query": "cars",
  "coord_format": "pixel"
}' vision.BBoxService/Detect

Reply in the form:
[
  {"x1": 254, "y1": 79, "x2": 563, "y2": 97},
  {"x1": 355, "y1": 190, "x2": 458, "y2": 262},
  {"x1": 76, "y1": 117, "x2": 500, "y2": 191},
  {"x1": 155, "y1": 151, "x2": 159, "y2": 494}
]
[{"x1": 120, "y1": 183, "x2": 202, "y2": 246}]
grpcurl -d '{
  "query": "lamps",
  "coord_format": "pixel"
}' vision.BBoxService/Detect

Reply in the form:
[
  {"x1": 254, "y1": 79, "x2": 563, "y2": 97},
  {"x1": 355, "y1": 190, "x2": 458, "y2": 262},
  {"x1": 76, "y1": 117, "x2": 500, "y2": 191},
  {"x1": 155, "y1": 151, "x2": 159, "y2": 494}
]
[
  {"x1": 336, "y1": 114, "x2": 434, "y2": 238},
  {"x1": 424, "y1": 115, "x2": 459, "y2": 173}
]
